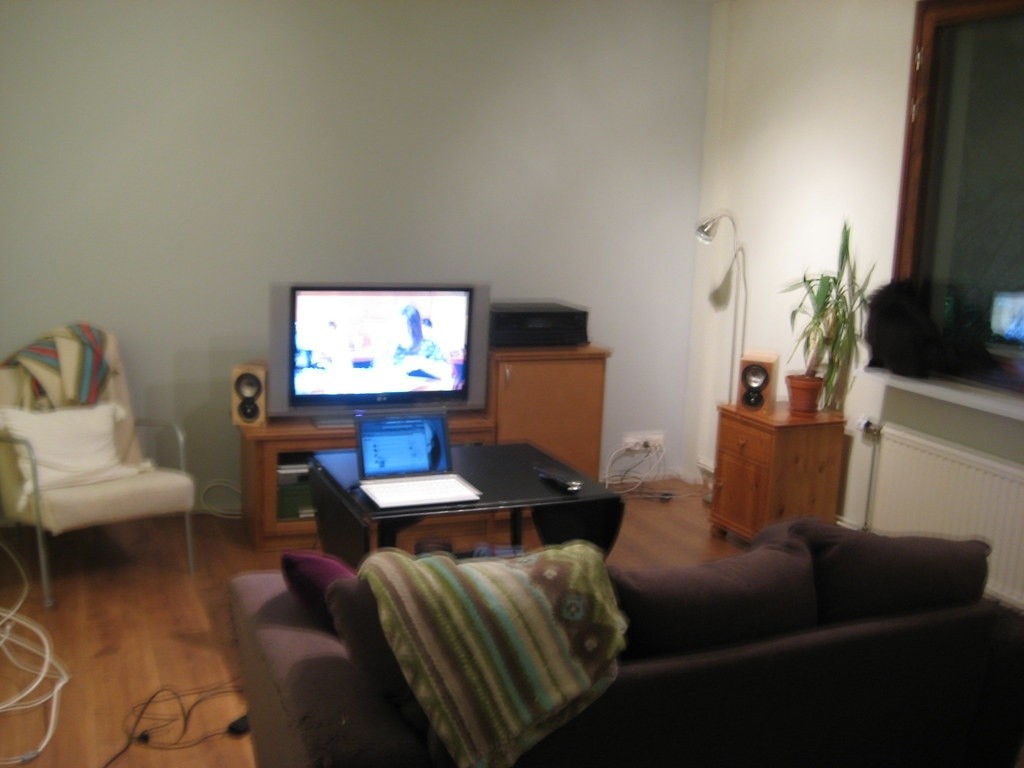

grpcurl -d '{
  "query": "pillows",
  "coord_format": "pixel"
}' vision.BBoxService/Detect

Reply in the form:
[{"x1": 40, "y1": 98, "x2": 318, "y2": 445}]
[
  {"x1": 336, "y1": 570, "x2": 420, "y2": 725},
  {"x1": 282, "y1": 552, "x2": 355, "y2": 625},
  {"x1": 0, "y1": 401, "x2": 157, "y2": 511},
  {"x1": 791, "y1": 516, "x2": 994, "y2": 620},
  {"x1": 607, "y1": 539, "x2": 817, "y2": 663}
]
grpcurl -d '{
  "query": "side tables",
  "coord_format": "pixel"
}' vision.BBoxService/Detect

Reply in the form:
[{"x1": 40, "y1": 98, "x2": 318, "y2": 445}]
[{"x1": 708, "y1": 404, "x2": 847, "y2": 544}]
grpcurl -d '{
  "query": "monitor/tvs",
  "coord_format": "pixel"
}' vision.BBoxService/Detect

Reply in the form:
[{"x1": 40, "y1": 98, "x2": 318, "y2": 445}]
[
  {"x1": 990, "y1": 293, "x2": 1024, "y2": 345},
  {"x1": 287, "y1": 286, "x2": 488, "y2": 429}
]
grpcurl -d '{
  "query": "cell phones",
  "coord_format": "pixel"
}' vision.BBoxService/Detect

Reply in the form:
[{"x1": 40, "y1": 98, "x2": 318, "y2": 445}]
[{"x1": 539, "y1": 469, "x2": 582, "y2": 492}]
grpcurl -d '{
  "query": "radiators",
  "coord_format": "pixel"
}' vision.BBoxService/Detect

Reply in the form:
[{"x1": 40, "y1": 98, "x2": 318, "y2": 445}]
[{"x1": 857, "y1": 416, "x2": 1024, "y2": 616}]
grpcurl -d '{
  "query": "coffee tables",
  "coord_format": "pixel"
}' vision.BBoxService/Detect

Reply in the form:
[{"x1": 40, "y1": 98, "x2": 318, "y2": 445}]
[{"x1": 308, "y1": 441, "x2": 625, "y2": 572}]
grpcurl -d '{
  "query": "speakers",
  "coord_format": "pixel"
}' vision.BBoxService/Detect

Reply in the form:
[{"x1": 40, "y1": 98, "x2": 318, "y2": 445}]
[
  {"x1": 738, "y1": 352, "x2": 780, "y2": 414},
  {"x1": 230, "y1": 364, "x2": 268, "y2": 428}
]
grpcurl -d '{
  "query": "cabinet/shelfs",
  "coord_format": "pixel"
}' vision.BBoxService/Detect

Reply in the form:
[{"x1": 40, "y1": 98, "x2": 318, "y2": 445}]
[{"x1": 238, "y1": 346, "x2": 614, "y2": 550}]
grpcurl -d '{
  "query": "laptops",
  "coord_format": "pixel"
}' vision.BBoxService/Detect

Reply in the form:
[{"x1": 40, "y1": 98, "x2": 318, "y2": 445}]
[{"x1": 353, "y1": 412, "x2": 483, "y2": 507}]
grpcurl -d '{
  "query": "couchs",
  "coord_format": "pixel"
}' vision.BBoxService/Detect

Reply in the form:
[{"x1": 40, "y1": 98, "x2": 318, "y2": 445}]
[{"x1": 224, "y1": 571, "x2": 1024, "y2": 768}]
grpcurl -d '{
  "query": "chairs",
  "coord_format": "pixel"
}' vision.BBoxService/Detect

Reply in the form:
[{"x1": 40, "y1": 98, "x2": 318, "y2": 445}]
[{"x1": 0, "y1": 327, "x2": 197, "y2": 615}]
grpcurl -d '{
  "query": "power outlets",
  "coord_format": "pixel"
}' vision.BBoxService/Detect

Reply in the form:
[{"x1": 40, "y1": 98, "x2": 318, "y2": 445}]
[{"x1": 621, "y1": 431, "x2": 664, "y2": 452}]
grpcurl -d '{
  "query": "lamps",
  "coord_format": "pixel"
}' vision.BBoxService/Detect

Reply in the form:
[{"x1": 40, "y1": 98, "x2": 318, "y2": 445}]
[{"x1": 696, "y1": 202, "x2": 741, "y2": 503}]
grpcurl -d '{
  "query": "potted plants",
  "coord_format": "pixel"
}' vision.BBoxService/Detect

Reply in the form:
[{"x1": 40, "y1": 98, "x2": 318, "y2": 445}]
[{"x1": 779, "y1": 222, "x2": 868, "y2": 419}]
